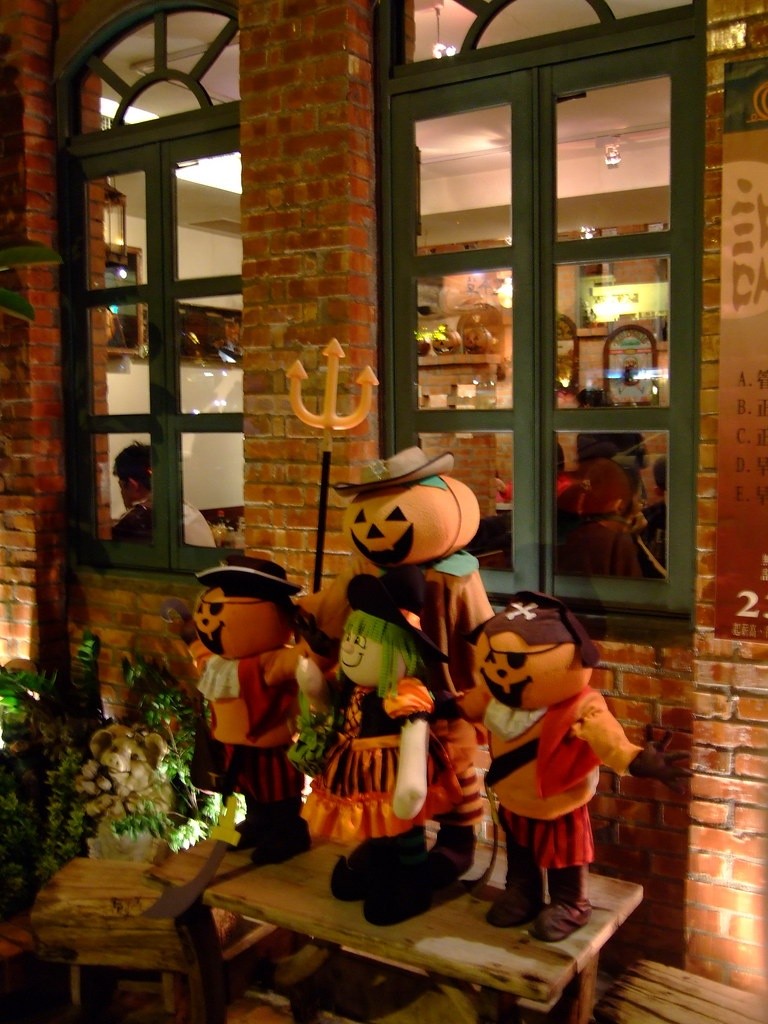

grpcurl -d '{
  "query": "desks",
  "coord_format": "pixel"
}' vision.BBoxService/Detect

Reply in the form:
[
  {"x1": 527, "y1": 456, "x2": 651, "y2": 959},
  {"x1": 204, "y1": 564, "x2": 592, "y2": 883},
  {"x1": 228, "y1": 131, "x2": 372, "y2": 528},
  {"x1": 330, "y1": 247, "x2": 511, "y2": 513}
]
[{"x1": 203, "y1": 832, "x2": 644, "y2": 1024}]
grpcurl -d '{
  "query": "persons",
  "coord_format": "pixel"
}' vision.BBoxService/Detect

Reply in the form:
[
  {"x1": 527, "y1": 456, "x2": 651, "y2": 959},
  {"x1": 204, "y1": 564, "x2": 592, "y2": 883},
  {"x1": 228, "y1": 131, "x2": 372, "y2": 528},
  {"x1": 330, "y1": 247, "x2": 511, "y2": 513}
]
[
  {"x1": 287, "y1": 564, "x2": 450, "y2": 924},
  {"x1": 553, "y1": 429, "x2": 669, "y2": 580},
  {"x1": 112, "y1": 444, "x2": 218, "y2": 547}
]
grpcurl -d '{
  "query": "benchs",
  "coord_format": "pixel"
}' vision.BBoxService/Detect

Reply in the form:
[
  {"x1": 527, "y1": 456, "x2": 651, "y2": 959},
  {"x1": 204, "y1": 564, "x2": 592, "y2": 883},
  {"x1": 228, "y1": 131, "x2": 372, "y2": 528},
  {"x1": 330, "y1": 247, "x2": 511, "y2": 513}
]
[{"x1": 593, "y1": 959, "x2": 767, "y2": 1024}]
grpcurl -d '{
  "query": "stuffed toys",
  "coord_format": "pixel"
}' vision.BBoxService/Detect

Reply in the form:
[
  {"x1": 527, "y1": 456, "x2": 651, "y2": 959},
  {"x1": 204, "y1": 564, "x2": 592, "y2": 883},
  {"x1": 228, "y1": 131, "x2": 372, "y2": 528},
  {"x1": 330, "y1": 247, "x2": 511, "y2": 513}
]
[
  {"x1": 287, "y1": 446, "x2": 495, "y2": 886},
  {"x1": 90, "y1": 726, "x2": 174, "y2": 814},
  {"x1": 468, "y1": 590, "x2": 693, "y2": 941},
  {"x1": 193, "y1": 556, "x2": 340, "y2": 863}
]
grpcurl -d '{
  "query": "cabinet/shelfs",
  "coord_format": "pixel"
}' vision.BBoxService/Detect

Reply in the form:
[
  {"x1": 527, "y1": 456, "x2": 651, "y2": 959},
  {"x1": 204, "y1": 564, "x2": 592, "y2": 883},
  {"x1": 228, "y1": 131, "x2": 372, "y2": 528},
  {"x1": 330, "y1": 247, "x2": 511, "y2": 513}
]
[{"x1": 105, "y1": 242, "x2": 144, "y2": 355}]
